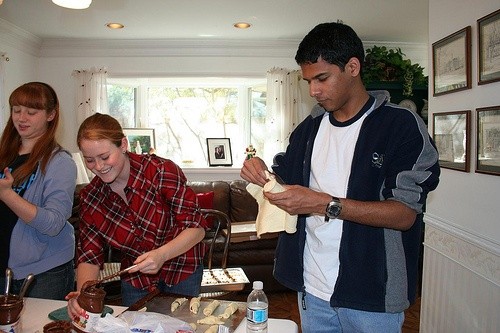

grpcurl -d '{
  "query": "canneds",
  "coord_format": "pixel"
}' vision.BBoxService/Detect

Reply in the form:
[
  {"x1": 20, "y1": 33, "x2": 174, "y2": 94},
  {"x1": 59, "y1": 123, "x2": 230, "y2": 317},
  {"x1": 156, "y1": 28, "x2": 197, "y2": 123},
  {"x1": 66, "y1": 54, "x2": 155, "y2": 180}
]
[
  {"x1": 0, "y1": 294, "x2": 24, "y2": 333},
  {"x1": 68, "y1": 287, "x2": 106, "y2": 333}
]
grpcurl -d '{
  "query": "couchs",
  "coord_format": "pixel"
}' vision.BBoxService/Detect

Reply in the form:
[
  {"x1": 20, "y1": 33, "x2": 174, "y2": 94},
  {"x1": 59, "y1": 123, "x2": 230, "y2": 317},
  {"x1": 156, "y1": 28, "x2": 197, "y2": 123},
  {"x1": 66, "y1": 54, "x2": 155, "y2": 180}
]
[{"x1": 67, "y1": 180, "x2": 279, "y2": 282}]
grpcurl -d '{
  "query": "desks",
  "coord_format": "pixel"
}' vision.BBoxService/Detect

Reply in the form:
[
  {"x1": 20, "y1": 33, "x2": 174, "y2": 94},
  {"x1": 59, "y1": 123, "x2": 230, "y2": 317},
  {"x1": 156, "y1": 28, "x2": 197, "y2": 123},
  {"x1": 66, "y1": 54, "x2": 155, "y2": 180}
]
[{"x1": 17, "y1": 298, "x2": 298, "y2": 333}]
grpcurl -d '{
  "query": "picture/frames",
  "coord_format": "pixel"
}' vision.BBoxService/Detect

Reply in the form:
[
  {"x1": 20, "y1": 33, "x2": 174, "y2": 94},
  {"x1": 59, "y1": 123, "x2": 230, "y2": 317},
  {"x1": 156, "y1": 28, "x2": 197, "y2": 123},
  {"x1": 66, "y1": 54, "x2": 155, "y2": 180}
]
[
  {"x1": 477, "y1": 9, "x2": 500, "y2": 85},
  {"x1": 432, "y1": 110, "x2": 471, "y2": 173},
  {"x1": 475, "y1": 106, "x2": 500, "y2": 176},
  {"x1": 123, "y1": 128, "x2": 156, "y2": 155},
  {"x1": 432, "y1": 26, "x2": 472, "y2": 97},
  {"x1": 206, "y1": 138, "x2": 233, "y2": 167}
]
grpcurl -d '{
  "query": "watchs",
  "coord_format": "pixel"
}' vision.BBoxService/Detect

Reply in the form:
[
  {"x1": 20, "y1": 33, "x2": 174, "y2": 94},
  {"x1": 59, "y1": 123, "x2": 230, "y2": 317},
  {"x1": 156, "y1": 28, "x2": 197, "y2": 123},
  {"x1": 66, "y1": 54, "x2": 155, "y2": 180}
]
[{"x1": 325, "y1": 197, "x2": 342, "y2": 222}]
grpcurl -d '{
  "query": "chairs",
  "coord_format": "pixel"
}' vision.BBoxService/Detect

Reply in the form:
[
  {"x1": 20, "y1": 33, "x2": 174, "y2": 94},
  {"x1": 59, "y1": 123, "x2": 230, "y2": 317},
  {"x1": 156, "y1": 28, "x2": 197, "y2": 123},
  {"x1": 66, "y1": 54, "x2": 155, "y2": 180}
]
[{"x1": 197, "y1": 208, "x2": 244, "y2": 292}]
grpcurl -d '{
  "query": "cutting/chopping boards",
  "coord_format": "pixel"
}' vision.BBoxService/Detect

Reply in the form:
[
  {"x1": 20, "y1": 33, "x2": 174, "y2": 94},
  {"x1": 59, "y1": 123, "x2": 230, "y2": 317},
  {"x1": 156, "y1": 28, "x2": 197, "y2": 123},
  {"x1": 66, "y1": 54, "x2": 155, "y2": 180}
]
[{"x1": 114, "y1": 289, "x2": 246, "y2": 333}]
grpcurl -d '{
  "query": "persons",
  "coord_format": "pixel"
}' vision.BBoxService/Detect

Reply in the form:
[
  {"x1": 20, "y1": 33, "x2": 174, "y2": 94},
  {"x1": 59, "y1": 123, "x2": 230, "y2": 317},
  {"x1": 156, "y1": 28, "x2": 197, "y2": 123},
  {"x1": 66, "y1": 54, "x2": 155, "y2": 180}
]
[
  {"x1": 65, "y1": 113, "x2": 208, "y2": 321},
  {"x1": 215, "y1": 145, "x2": 224, "y2": 159},
  {"x1": 239, "y1": 22, "x2": 441, "y2": 333},
  {"x1": 0, "y1": 81, "x2": 77, "y2": 301}
]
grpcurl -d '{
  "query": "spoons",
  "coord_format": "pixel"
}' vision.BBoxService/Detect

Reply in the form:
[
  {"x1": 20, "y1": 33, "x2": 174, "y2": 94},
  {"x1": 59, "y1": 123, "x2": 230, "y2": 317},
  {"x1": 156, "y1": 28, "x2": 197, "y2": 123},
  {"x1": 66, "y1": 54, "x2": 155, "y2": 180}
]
[{"x1": 84, "y1": 262, "x2": 143, "y2": 289}]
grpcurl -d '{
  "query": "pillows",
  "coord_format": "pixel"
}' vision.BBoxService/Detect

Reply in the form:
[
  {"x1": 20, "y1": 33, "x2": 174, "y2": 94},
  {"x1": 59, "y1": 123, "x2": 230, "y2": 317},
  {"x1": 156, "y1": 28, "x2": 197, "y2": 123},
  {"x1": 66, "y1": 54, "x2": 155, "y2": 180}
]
[{"x1": 197, "y1": 191, "x2": 215, "y2": 230}]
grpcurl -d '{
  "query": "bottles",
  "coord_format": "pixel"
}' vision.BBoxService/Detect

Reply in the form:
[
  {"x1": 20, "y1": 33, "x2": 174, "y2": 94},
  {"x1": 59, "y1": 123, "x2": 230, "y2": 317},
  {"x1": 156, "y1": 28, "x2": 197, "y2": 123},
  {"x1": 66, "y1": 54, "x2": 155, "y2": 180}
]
[
  {"x1": 44, "y1": 320, "x2": 71, "y2": 333},
  {"x1": 246, "y1": 281, "x2": 268, "y2": 333},
  {"x1": 71, "y1": 288, "x2": 106, "y2": 333},
  {"x1": 0, "y1": 295, "x2": 23, "y2": 333}
]
